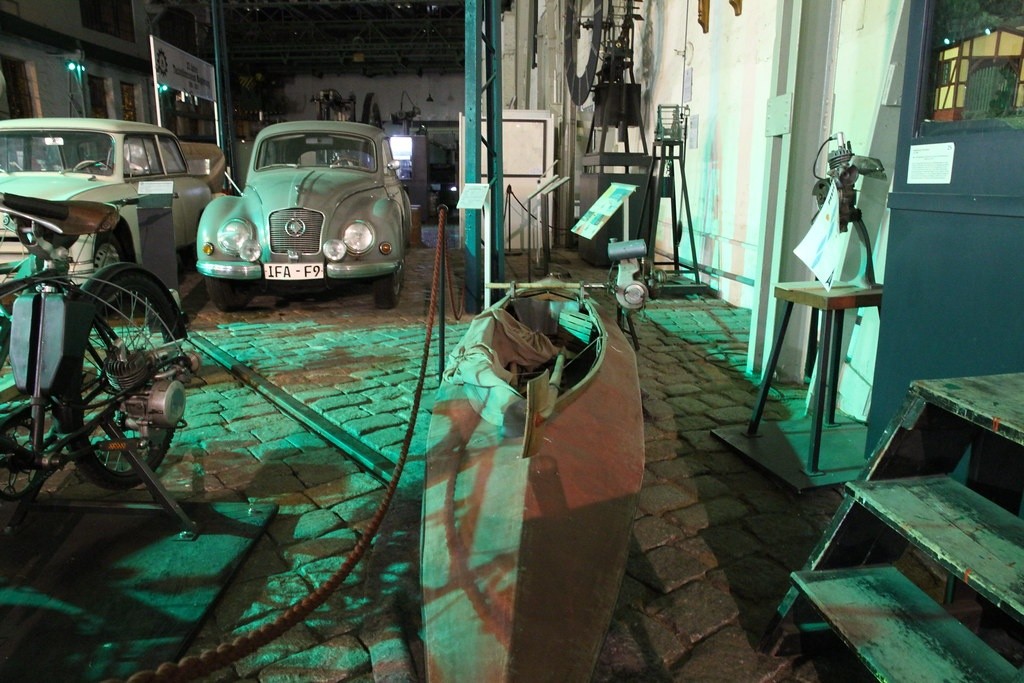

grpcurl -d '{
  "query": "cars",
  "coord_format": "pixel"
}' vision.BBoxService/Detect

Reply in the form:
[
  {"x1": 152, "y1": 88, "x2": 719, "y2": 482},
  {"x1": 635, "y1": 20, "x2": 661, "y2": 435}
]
[
  {"x1": 196, "y1": 120, "x2": 411, "y2": 312},
  {"x1": 0, "y1": 116, "x2": 212, "y2": 297}
]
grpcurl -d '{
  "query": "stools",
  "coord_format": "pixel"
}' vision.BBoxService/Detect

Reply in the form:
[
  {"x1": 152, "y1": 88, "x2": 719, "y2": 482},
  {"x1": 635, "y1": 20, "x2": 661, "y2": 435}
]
[{"x1": 746, "y1": 282, "x2": 885, "y2": 476}]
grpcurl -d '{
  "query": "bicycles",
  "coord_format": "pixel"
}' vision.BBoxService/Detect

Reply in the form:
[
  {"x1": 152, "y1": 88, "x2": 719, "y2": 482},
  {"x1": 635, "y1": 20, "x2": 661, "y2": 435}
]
[{"x1": 0, "y1": 192, "x2": 203, "y2": 542}]
